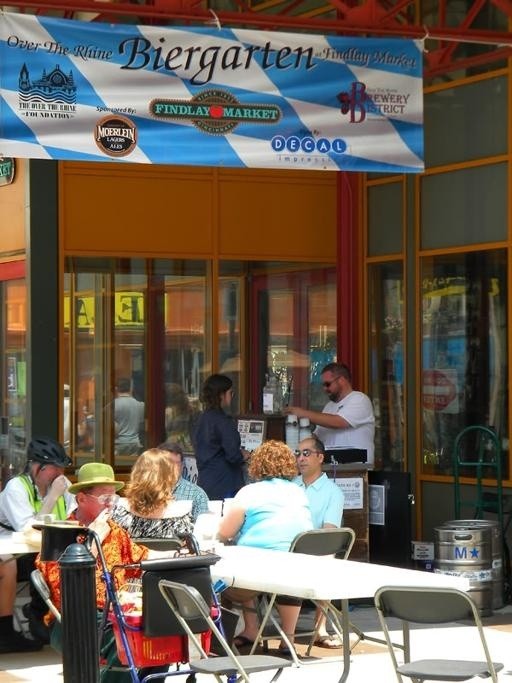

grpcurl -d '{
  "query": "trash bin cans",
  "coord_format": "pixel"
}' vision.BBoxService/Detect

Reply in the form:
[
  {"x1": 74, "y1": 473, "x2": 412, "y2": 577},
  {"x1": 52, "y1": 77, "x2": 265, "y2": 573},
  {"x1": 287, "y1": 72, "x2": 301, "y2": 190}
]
[{"x1": 432, "y1": 519, "x2": 505, "y2": 619}]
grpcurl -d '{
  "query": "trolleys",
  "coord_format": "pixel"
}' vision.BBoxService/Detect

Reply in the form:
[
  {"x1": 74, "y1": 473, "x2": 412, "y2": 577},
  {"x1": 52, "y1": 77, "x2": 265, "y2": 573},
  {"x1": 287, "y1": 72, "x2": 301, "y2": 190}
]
[
  {"x1": 86, "y1": 528, "x2": 239, "y2": 683},
  {"x1": 451, "y1": 423, "x2": 512, "y2": 603}
]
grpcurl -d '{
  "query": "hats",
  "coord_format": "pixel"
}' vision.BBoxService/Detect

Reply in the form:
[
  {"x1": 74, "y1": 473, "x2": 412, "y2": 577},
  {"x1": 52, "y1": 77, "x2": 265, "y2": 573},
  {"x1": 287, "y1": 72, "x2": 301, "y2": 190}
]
[{"x1": 68, "y1": 462, "x2": 123, "y2": 493}]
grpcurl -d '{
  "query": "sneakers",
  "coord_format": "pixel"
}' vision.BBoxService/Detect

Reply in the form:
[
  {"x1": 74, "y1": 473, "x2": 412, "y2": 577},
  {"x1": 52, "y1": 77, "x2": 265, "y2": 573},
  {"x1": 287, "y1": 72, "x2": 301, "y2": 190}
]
[{"x1": 0, "y1": 609, "x2": 54, "y2": 653}]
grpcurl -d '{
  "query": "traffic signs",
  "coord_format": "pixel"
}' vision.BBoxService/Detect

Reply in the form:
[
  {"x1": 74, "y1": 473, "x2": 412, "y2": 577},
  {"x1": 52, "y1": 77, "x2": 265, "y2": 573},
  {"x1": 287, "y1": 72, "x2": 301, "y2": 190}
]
[{"x1": 420, "y1": 368, "x2": 459, "y2": 416}]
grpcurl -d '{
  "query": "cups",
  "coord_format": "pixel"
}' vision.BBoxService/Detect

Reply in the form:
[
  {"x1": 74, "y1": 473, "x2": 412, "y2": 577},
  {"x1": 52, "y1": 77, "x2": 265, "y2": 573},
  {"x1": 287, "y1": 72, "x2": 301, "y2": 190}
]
[{"x1": 285, "y1": 415, "x2": 311, "y2": 445}]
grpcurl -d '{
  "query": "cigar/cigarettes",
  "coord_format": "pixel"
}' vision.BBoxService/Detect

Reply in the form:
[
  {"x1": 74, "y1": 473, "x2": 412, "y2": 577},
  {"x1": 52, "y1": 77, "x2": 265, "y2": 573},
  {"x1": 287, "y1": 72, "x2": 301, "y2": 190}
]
[{"x1": 104, "y1": 512, "x2": 110, "y2": 514}]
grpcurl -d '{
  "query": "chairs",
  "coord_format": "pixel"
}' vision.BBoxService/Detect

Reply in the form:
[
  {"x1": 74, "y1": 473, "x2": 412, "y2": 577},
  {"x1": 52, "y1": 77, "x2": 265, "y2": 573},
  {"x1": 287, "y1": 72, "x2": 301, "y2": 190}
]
[
  {"x1": 156, "y1": 580, "x2": 293, "y2": 682},
  {"x1": 28, "y1": 570, "x2": 143, "y2": 683},
  {"x1": 373, "y1": 586, "x2": 504, "y2": 683},
  {"x1": 248, "y1": 527, "x2": 364, "y2": 669},
  {"x1": 125, "y1": 533, "x2": 185, "y2": 557}
]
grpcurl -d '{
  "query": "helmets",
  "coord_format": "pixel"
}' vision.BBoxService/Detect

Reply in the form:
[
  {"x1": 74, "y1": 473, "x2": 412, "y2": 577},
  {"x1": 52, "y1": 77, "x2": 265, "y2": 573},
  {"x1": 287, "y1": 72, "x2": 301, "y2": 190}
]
[{"x1": 26, "y1": 437, "x2": 73, "y2": 468}]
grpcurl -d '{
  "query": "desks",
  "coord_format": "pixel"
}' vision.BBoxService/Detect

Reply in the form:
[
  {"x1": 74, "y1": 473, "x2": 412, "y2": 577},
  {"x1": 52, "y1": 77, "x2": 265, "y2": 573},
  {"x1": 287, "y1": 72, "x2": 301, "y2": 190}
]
[
  {"x1": 199, "y1": 545, "x2": 470, "y2": 683},
  {"x1": 1, "y1": 529, "x2": 43, "y2": 556}
]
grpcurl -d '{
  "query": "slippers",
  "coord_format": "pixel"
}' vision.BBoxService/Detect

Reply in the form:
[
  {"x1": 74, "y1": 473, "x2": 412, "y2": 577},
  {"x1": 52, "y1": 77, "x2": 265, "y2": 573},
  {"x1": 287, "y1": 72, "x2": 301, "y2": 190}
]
[
  {"x1": 230, "y1": 632, "x2": 262, "y2": 648},
  {"x1": 309, "y1": 633, "x2": 342, "y2": 649},
  {"x1": 278, "y1": 642, "x2": 297, "y2": 655}
]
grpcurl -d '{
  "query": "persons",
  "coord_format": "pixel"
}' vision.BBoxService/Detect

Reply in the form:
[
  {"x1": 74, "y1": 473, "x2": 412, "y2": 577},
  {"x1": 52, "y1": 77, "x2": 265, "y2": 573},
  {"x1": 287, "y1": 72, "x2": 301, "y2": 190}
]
[
  {"x1": 103, "y1": 448, "x2": 198, "y2": 551},
  {"x1": 1, "y1": 436, "x2": 81, "y2": 645},
  {"x1": 209, "y1": 438, "x2": 318, "y2": 655},
  {"x1": 154, "y1": 441, "x2": 206, "y2": 522},
  {"x1": 192, "y1": 374, "x2": 248, "y2": 499},
  {"x1": 1, "y1": 551, "x2": 44, "y2": 656},
  {"x1": 32, "y1": 459, "x2": 170, "y2": 683},
  {"x1": 101, "y1": 376, "x2": 146, "y2": 453},
  {"x1": 284, "y1": 362, "x2": 377, "y2": 469},
  {"x1": 283, "y1": 436, "x2": 348, "y2": 650},
  {"x1": 165, "y1": 383, "x2": 195, "y2": 447}
]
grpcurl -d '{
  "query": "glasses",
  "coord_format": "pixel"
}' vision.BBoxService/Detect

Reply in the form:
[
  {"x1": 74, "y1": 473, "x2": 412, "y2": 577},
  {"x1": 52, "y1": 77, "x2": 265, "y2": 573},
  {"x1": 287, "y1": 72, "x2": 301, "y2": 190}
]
[
  {"x1": 321, "y1": 374, "x2": 344, "y2": 387},
  {"x1": 294, "y1": 449, "x2": 324, "y2": 458},
  {"x1": 84, "y1": 490, "x2": 118, "y2": 505}
]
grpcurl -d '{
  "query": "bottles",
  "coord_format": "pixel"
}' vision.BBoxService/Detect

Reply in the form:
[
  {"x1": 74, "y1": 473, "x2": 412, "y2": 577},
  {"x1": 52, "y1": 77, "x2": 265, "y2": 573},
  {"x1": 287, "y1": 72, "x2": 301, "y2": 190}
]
[{"x1": 263, "y1": 376, "x2": 285, "y2": 415}]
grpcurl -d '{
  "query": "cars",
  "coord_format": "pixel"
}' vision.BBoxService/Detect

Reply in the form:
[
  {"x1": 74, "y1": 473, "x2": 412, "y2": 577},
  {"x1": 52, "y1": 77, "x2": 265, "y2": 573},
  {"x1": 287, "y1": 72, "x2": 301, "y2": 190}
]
[{"x1": 0, "y1": 390, "x2": 72, "y2": 458}]
